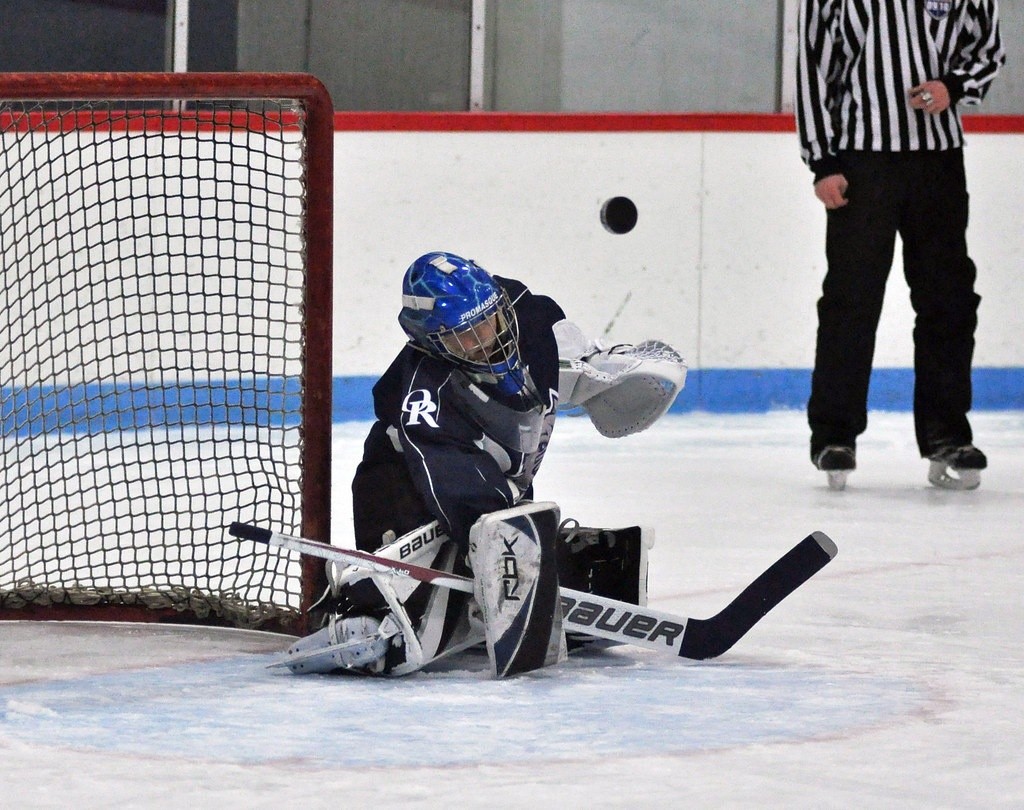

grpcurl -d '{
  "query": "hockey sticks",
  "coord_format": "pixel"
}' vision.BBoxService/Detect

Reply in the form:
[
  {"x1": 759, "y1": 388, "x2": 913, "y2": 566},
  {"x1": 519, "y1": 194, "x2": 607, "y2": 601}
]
[{"x1": 223, "y1": 521, "x2": 841, "y2": 660}]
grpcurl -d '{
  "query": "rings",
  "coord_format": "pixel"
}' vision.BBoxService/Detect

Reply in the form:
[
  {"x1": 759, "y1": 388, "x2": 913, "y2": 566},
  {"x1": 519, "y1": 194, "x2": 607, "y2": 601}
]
[{"x1": 921, "y1": 91, "x2": 933, "y2": 105}]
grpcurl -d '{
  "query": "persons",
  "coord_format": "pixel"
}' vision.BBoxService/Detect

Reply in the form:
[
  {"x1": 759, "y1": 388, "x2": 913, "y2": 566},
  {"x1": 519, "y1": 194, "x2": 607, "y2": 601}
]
[
  {"x1": 265, "y1": 251, "x2": 687, "y2": 672},
  {"x1": 794, "y1": 0, "x2": 1007, "y2": 492}
]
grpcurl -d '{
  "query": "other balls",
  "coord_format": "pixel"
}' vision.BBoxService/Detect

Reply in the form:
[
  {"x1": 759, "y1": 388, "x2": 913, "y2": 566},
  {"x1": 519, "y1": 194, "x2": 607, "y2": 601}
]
[{"x1": 600, "y1": 196, "x2": 642, "y2": 235}]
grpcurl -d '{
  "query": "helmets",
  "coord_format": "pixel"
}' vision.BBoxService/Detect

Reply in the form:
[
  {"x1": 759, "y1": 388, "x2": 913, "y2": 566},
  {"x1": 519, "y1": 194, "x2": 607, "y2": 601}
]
[{"x1": 398, "y1": 251, "x2": 522, "y2": 376}]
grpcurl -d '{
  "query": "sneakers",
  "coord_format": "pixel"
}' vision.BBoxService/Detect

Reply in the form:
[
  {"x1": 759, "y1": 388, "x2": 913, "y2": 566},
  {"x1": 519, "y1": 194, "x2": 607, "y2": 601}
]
[
  {"x1": 809, "y1": 439, "x2": 856, "y2": 491},
  {"x1": 927, "y1": 444, "x2": 987, "y2": 491}
]
